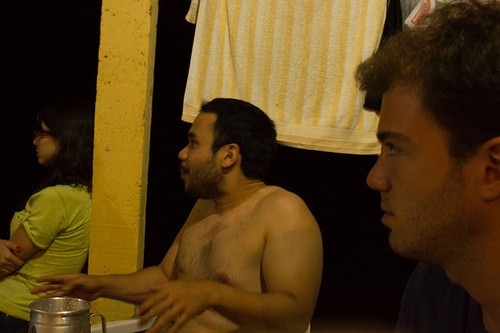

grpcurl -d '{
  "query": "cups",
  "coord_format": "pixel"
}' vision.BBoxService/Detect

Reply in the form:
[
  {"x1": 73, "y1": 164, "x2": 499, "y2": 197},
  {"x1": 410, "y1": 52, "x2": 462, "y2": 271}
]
[{"x1": 26, "y1": 296, "x2": 107, "y2": 333}]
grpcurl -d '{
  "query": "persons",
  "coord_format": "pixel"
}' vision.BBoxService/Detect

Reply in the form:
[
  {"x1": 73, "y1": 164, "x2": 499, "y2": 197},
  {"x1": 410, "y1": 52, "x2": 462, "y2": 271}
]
[
  {"x1": 0, "y1": 103, "x2": 96, "y2": 333},
  {"x1": 27, "y1": 96, "x2": 324, "y2": 333},
  {"x1": 354, "y1": 0, "x2": 499, "y2": 333}
]
